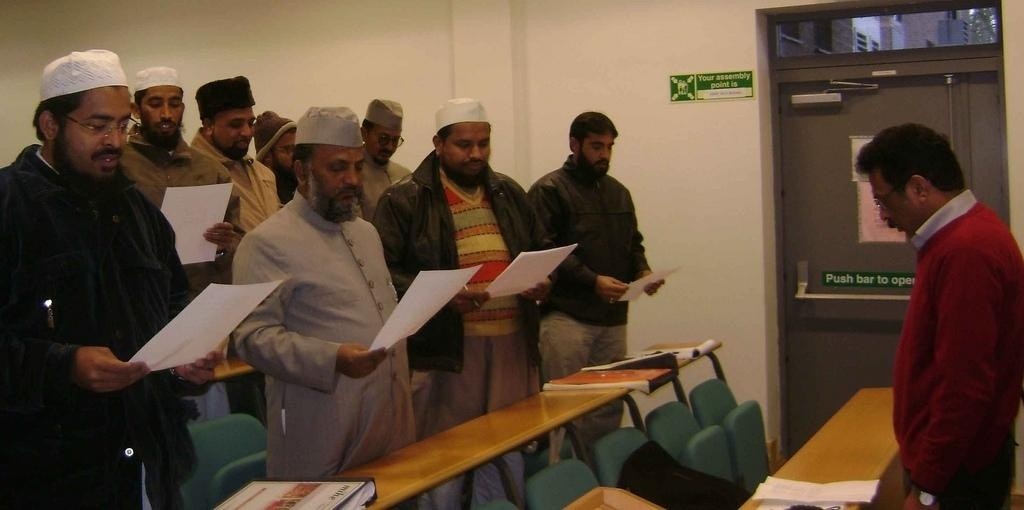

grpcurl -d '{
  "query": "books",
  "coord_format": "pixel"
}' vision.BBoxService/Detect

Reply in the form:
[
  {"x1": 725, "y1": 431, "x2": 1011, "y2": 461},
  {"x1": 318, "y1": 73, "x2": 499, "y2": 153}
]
[
  {"x1": 212, "y1": 478, "x2": 377, "y2": 510},
  {"x1": 540, "y1": 366, "x2": 675, "y2": 396},
  {"x1": 625, "y1": 338, "x2": 715, "y2": 360}
]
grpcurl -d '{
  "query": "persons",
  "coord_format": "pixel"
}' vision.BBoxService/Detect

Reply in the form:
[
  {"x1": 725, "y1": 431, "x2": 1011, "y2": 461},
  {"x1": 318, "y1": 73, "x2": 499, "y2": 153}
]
[
  {"x1": 843, "y1": 117, "x2": 1023, "y2": 510},
  {"x1": 0, "y1": 46, "x2": 228, "y2": 508},
  {"x1": 524, "y1": 108, "x2": 661, "y2": 463},
  {"x1": 233, "y1": 105, "x2": 416, "y2": 482},
  {"x1": 352, "y1": 100, "x2": 552, "y2": 436},
  {"x1": 120, "y1": 68, "x2": 298, "y2": 290}
]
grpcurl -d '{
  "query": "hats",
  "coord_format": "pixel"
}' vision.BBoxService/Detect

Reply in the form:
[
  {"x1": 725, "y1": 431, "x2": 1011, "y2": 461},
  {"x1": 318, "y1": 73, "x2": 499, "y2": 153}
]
[
  {"x1": 294, "y1": 105, "x2": 365, "y2": 149},
  {"x1": 194, "y1": 75, "x2": 256, "y2": 121},
  {"x1": 365, "y1": 96, "x2": 404, "y2": 132},
  {"x1": 133, "y1": 65, "x2": 185, "y2": 96},
  {"x1": 434, "y1": 97, "x2": 492, "y2": 136},
  {"x1": 253, "y1": 110, "x2": 297, "y2": 163},
  {"x1": 37, "y1": 47, "x2": 130, "y2": 104}
]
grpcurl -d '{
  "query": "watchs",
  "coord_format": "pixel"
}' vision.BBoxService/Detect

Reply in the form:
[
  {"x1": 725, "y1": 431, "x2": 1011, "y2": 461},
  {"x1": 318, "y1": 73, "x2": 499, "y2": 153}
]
[{"x1": 909, "y1": 483, "x2": 942, "y2": 508}]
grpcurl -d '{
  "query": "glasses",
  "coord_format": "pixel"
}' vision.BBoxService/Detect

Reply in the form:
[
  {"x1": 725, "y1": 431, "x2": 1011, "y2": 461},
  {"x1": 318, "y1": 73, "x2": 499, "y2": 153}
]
[
  {"x1": 272, "y1": 145, "x2": 295, "y2": 159},
  {"x1": 64, "y1": 112, "x2": 142, "y2": 139},
  {"x1": 367, "y1": 130, "x2": 405, "y2": 149},
  {"x1": 871, "y1": 187, "x2": 898, "y2": 213}
]
